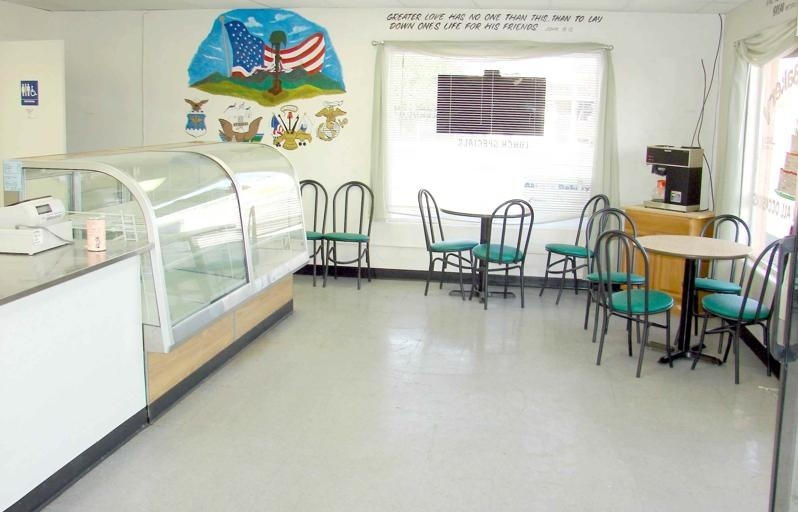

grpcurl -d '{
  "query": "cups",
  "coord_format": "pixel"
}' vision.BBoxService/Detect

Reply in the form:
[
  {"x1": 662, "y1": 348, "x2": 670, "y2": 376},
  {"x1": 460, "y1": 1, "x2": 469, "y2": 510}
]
[{"x1": 86, "y1": 219, "x2": 106, "y2": 251}]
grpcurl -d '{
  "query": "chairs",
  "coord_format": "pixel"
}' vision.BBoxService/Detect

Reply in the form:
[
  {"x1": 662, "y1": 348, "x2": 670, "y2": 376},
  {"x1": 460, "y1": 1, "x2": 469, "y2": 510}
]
[
  {"x1": 589, "y1": 229, "x2": 675, "y2": 377},
  {"x1": 469, "y1": 198, "x2": 539, "y2": 311},
  {"x1": 583, "y1": 208, "x2": 649, "y2": 342},
  {"x1": 322, "y1": 178, "x2": 378, "y2": 291},
  {"x1": 689, "y1": 236, "x2": 794, "y2": 386},
  {"x1": 539, "y1": 193, "x2": 614, "y2": 305},
  {"x1": 414, "y1": 184, "x2": 481, "y2": 303},
  {"x1": 674, "y1": 214, "x2": 753, "y2": 356},
  {"x1": 295, "y1": 178, "x2": 330, "y2": 289}
]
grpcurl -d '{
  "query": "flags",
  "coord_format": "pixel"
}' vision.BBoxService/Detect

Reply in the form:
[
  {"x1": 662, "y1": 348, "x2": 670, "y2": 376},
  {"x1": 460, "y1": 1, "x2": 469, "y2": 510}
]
[
  {"x1": 224, "y1": 21, "x2": 266, "y2": 79},
  {"x1": 262, "y1": 32, "x2": 325, "y2": 74}
]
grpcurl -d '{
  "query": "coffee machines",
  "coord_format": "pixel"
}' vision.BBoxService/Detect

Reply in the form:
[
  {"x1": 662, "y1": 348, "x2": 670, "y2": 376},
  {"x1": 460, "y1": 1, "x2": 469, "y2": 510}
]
[{"x1": 643, "y1": 144, "x2": 705, "y2": 212}]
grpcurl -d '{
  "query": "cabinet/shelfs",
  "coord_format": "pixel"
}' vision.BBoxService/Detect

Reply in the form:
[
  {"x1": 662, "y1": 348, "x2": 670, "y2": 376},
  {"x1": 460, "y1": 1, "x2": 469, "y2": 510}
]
[
  {"x1": 3, "y1": 142, "x2": 309, "y2": 426},
  {"x1": 620, "y1": 205, "x2": 718, "y2": 317}
]
